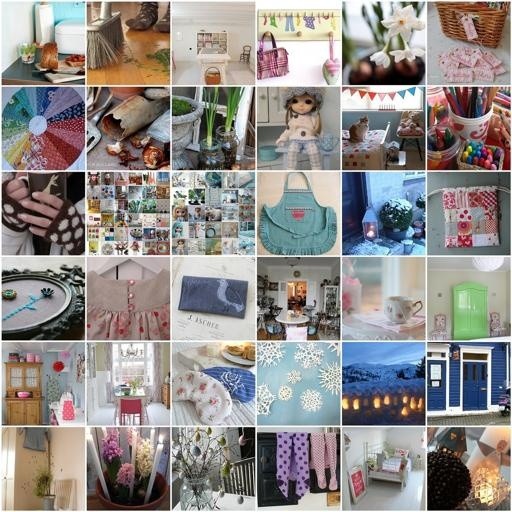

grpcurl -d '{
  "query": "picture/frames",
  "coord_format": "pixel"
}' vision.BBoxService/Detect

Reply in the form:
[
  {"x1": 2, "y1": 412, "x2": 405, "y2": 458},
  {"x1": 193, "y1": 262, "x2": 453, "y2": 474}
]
[{"x1": 269, "y1": 282, "x2": 279, "y2": 291}]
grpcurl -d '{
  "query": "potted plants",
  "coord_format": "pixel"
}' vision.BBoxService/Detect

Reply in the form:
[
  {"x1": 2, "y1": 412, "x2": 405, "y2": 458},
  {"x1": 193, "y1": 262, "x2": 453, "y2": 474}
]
[
  {"x1": 380, "y1": 198, "x2": 414, "y2": 240},
  {"x1": 172, "y1": 428, "x2": 247, "y2": 511},
  {"x1": 21, "y1": 454, "x2": 63, "y2": 509},
  {"x1": 126, "y1": 380, "x2": 142, "y2": 393},
  {"x1": 172, "y1": 87, "x2": 245, "y2": 170}
]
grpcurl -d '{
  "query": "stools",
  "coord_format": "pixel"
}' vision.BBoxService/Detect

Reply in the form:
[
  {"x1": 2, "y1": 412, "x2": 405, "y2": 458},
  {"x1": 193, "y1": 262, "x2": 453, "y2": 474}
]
[{"x1": 386, "y1": 151, "x2": 406, "y2": 170}]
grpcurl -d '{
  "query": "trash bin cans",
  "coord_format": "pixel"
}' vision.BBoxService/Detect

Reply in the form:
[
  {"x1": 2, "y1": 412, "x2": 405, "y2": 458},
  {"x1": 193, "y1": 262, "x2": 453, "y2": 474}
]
[
  {"x1": 414, "y1": 221, "x2": 424, "y2": 238},
  {"x1": 42, "y1": 494, "x2": 55, "y2": 510}
]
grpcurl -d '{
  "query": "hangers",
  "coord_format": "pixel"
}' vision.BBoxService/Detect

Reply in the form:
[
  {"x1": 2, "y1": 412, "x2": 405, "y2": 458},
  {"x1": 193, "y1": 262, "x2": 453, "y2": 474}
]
[{"x1": 96, "y1": 257, "x2": 162, "y2": 277}]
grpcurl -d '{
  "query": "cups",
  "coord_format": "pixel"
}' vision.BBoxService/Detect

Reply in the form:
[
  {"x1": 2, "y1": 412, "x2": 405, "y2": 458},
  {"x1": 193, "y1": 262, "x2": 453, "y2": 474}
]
[
  {"x1": 384, "y1": 297, "x2": 424, "y2": 322},
  {"x1": 428, "y1": 87, "x2": 490, "y2": 167},
  {"x1": 18, "y1": 43, "x2": 36, "y2": 64}
]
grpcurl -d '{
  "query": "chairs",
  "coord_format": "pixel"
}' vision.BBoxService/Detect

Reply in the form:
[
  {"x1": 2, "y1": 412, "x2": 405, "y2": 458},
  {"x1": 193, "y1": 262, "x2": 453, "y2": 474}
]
[
  {"x1": 307, "y1": 309, "x2": 336, "y2": 340},
  {"x1": 431, "y1": 314, "x2": 447, "y2": 340},
  {"x1": 490, "y1": 313, "x2": 506, "y2": 337},
  {"x1": 397, "y1": 111, "x2": 423, "y2": 163},
  {"x1": 120, "y1": 399, "x2": 142, "y2": 426},
  {"x1": 239, "y1": 45, "x2": 251, "y2": 64}
]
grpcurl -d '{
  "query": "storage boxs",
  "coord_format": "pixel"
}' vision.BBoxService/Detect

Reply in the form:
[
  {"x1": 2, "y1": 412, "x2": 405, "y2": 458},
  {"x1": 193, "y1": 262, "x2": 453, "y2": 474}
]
[{"x1": 54, "y1": 20, "x2": 86, "y2": 55}]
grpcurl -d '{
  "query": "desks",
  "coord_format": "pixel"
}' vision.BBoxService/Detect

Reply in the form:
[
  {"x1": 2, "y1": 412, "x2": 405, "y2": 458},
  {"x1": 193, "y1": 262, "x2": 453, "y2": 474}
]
[
  {"x1": 114, "y1": 388, "x2": 146, "y2": 426},
  {"x1": 257, "y1": 306, "x2": 310, "y2": 339},
  {"x1": 198, "y1": 48, "x2": 231, "y2": 70}
]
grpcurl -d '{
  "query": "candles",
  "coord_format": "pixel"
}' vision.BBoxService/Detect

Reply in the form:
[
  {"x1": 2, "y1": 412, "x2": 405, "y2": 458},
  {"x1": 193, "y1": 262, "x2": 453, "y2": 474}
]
[{"x1": 86, "y1": 426, "x2": 164, "y2": 504}]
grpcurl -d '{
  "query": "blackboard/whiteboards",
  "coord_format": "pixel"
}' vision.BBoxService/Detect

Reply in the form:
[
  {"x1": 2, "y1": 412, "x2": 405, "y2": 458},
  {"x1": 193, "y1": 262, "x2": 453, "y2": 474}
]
[{"x1": 349, "y1": 465, "x2": 367, "y2": 504}]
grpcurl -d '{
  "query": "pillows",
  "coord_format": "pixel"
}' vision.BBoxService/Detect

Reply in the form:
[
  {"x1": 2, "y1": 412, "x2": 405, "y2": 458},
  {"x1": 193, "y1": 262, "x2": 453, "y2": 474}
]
[{"x1": 393, "y1": 448, "x2": 408, "y2": 460}]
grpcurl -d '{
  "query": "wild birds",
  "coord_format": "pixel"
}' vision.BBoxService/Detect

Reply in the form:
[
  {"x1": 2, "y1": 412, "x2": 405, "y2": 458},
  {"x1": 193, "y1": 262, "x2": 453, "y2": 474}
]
[{"x1": 215, "y1": 279, "x2": 245, "y2": 315}]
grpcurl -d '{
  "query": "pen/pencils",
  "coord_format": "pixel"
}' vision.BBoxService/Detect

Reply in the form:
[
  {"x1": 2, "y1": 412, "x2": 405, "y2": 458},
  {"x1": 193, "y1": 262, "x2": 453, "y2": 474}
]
[{"x1": 442, "y1": 87, "x2": 498, "y2": 118}]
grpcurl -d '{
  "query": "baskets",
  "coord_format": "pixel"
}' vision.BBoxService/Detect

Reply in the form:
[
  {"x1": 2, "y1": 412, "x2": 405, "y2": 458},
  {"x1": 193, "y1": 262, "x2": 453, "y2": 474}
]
[
  {"x1": 204, "y1": 67, "x2": 221, "y2": 85},
  {"x1": 435, "y1": 3, "x2": 510, "y2": 49}
]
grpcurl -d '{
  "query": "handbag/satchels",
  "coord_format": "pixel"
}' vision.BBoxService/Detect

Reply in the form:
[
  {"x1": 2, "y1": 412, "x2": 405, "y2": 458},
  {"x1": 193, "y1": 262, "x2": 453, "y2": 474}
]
[{"x1": 257, "y1": 31, "x2": 289, "y2": 80}]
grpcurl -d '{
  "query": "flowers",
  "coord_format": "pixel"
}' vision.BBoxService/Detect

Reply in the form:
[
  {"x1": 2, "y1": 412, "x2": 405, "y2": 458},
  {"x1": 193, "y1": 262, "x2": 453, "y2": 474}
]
[{"x1": 342, "y1": 1, "x2": 425, "y2": 83}]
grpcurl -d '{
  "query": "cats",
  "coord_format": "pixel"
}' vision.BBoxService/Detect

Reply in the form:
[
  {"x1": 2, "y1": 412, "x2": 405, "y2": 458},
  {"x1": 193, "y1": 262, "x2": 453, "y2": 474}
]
[{"x1": 342, "y1": 115, "x2": 370, "y2": 144}]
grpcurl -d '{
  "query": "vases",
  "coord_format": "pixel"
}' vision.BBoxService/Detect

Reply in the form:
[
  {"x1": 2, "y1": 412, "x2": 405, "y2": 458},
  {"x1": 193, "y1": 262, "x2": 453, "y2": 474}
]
[
  {"x1": 96, "y1": 468, "x2": 168, "y2": 510},
  {"x1": 342, "y1": 47, "x2": 426, "y2": 85}
]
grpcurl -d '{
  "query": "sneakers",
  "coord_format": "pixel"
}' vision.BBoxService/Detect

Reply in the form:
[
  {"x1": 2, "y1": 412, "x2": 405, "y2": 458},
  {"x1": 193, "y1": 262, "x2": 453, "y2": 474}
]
[
  {"x1": 124, "y1": 3, "x2": 158, "y2": 30},
  {"x1": 153, "y1": 3, "x2": 170, "y2": 33}
]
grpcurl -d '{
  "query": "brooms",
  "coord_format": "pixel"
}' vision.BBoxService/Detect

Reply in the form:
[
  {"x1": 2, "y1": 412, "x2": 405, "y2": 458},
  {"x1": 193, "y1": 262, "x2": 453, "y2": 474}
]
[{"x1": 87, "y1": 2, "x2": 126, "y2": 70}]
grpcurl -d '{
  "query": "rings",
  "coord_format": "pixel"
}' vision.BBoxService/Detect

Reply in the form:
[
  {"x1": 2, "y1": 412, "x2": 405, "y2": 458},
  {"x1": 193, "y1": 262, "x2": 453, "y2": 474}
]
[{"x1": 46, "y1": 219, "x2": 53, "y2": 227}]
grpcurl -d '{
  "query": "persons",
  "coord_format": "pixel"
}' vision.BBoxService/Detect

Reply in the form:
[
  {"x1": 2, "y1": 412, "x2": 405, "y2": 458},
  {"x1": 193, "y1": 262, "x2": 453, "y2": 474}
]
[
  {"x1": 2, "y1": 173, "x2": 86, "y2": 255},
  {"x1": 297, "y1": 295, "x2": 304, "y2": 309},
  {"x1": 125, "y1": 2, "x2": 170, "y2": 34}
]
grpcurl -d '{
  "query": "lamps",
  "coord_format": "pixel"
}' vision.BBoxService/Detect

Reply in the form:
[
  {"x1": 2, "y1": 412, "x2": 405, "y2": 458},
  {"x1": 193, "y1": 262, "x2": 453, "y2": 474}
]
[
  {"x1": 285, "y1": 258, "x2": 301, "y2": 266},
  {"x1": 120, "y1": 343, "x2": 143, "y2": 358},
  {"x1": 362, "y1": 202, "x2": 379, "y2": 244}
]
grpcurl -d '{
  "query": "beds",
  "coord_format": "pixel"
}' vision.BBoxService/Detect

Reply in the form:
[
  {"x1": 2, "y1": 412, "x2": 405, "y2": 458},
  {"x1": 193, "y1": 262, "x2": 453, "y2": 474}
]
[
  {"x1": 343, "y1": 122, "x2": 391, "y2": 169},
  {"x1": 366, "y1": 456, "x2": 412, "y2": 491}
]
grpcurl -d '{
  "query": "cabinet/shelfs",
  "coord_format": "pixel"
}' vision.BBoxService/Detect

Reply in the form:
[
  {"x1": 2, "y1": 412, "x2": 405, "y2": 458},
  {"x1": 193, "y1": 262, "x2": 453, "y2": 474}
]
[
  {"x1": 3, "y1": 361, "x2": 43, "y2": 425},
  {"x1": 163, "y1": 383, "x2": 170, "y2": 409},
  {"x1": 196, "y1": 32, "x2": 228, "y2": 57},
  {"x1": 320, "y1": 279, "x2": 339, "y2": 313},
  {"x1": 452, "y1": 281, "x2": 488, "y2": 340}
]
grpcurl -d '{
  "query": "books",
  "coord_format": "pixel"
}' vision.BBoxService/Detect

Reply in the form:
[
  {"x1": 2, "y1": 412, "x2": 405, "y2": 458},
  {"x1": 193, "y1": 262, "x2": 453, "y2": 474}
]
[
  {"x1": 275, "y1": 87, "x2": 324, "y2": 169},
  {"x1": 36, "y1": 61, "x2": 86, "y2": 85},
  {"x1": 399, "y1": 112, "x2": 422, "y2": 129},
  {"x1": 197, "y1": 33, "x2": 226, "y2": 54}
]
